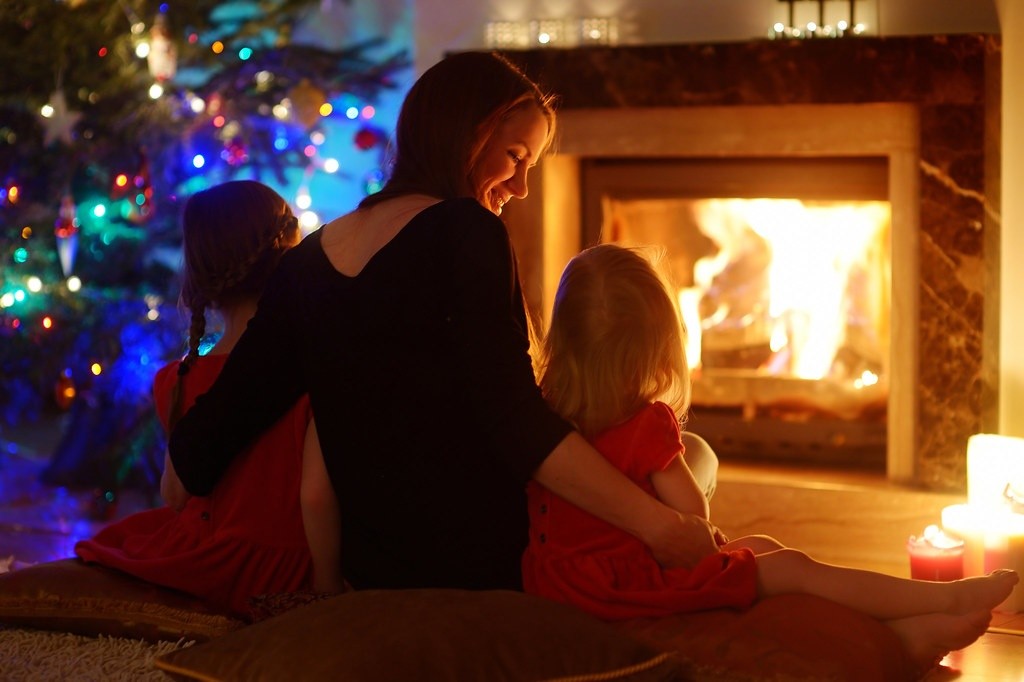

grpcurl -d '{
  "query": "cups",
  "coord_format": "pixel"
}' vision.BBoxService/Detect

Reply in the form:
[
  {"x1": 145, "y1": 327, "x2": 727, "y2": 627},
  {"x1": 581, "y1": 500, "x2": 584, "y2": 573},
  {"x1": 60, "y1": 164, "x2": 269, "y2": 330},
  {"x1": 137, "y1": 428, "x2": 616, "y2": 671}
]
[
  {"x1": 908, "y1": 535, "x2": 965, "y2": 581},
  {"x1": 943, "y1": 505, "x2": 986, "y2": 580}
]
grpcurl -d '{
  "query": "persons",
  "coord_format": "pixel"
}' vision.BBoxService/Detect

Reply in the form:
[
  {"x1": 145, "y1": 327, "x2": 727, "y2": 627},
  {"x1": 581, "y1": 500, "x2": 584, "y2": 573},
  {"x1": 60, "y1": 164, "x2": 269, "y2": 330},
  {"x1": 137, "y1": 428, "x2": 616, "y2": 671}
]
[
  {"x1": 74, "y1": 179, "x2": 340, "y2": 624},
  {"x1": 160, "y1": 52, "x2": 726, "y2": 592},
  {"x1": 521, "y1": 245, "x2": 1019, "y2": 671}
]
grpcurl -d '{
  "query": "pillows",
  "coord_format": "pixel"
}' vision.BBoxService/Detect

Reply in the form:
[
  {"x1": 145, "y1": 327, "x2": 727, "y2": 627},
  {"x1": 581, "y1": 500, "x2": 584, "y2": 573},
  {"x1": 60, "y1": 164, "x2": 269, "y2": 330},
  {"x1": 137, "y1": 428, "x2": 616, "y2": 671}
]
[
  {"x1": 0, "y1": 555, "x2": 245, "y2": 648},
  {"x1": 152, "y1": 592, "x2": 689, "y2": 682}
]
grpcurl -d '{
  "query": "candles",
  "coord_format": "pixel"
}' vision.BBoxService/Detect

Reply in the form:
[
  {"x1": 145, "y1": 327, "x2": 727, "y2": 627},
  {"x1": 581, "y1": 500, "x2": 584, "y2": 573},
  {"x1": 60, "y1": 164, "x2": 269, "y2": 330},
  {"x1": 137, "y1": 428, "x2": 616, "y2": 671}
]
[{"x1": 909, "y1": 524, "x2": 965, "y2": 581}]
